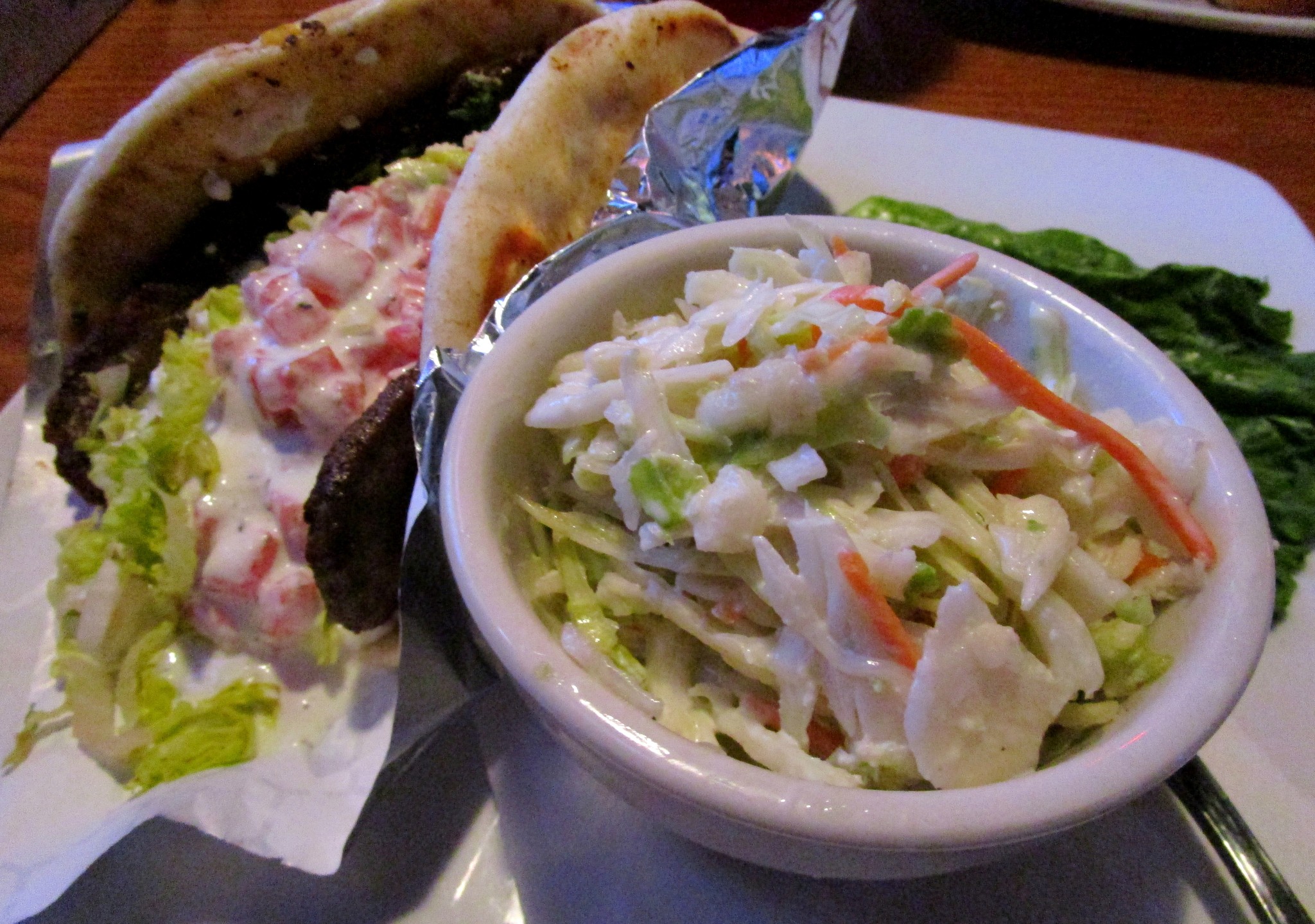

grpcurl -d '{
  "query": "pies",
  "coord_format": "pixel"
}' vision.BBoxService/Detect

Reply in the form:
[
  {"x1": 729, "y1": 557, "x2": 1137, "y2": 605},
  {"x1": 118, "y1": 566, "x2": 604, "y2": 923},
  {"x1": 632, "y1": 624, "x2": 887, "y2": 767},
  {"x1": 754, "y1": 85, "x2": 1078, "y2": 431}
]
[{"x1": 42, "y1": 1, "x2": 770, "y2": 794}]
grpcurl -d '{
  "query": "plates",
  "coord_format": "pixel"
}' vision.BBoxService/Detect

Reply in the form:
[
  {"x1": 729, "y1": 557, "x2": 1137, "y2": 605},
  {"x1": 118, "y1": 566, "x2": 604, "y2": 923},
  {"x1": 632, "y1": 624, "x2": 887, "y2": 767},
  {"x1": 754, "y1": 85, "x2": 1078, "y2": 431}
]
[
  {"x1": 1, "y1": 93, "x2": 1315, "y2": 921},
  {"x1": 1059, "y1": 0, "x2": 1315, "y2": 39}
]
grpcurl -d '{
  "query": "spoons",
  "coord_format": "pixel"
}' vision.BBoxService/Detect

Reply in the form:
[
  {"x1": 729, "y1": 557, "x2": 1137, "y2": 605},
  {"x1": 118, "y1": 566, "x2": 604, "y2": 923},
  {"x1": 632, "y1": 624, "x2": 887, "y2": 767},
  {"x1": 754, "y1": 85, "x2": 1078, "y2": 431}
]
[{"x1": 1164, "y1": 751, "x2": 1314, "y2": 924}]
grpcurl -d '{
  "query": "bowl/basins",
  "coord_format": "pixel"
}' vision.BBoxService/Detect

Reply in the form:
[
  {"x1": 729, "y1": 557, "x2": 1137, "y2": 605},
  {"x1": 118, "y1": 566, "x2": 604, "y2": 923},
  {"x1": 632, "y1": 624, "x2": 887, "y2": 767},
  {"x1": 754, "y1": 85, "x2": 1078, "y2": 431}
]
[{"x1": 441, "y1": 214, "x2": 1278, "y2": 889}]
